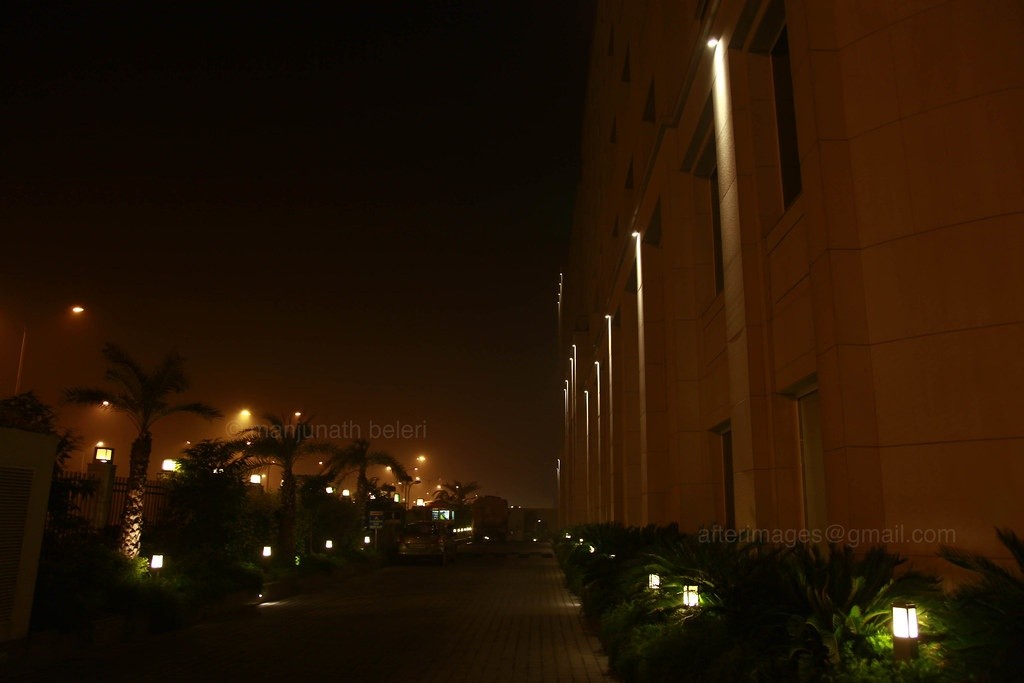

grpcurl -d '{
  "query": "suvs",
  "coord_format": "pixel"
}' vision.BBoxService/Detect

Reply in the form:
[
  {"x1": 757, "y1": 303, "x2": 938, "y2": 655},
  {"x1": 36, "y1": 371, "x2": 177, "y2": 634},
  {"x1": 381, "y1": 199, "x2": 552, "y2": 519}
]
[{"x1": 399, "y1": 520, "x2": 457, "y2": 568}]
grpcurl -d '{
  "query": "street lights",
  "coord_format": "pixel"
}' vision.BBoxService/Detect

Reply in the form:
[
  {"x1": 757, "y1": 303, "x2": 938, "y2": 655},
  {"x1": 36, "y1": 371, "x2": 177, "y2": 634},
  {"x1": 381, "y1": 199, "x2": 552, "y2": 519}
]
[{"x1": 407, "y1": 480, "x2": 422, "y2": 511}]
